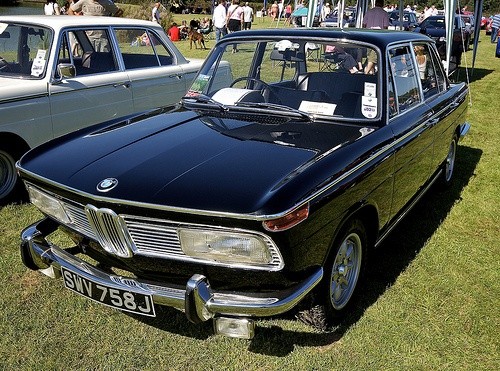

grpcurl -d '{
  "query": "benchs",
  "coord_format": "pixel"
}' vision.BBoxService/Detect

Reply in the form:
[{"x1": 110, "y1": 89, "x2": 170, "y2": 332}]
[
  {"x1": 296, "y1": 71, "x2": 435, "y2": 100},
  {"x1": 82, "y1": 52, "x2": 173, "y2": 73}
]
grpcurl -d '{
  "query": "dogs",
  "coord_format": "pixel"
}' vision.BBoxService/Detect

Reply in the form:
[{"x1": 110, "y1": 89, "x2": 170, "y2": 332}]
[{"x1": 181, "y1": 25, "x2": 207, "y2": 50}]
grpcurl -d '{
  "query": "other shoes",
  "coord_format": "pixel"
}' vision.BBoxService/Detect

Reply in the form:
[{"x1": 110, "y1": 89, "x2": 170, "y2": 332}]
[{"x1": 232, "y1": 49, "x2": 239, "y2": 53}]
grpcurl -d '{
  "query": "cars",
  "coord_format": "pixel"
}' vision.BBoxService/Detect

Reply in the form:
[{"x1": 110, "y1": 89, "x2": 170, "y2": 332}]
[
  {"x1": 14, "y1": 28, "x2": 474, "y2": 340},
  {"x1": 0, "y1": 15, "x2": 239, "y2": 207},
  {"x1": 319, "y1": 8, "x2": 363, "y2": 31},
  {"x1": 384, "y1": 11, "x2": 419, "y2": 31},
  {"x1": 414, "y1": 14, "x2": 476, "y2": 51}
]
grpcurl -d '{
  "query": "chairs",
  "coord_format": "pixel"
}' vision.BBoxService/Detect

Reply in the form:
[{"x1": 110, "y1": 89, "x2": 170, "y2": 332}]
[
  {"x1": 339, "y1": 92, "x2": 366, "y2": 118},
  {"x1": 272, "y1": 43, "x2": 339, "y2": 74},
  {"x1": 434, "y1": 38, "x2": 465, "y2": 83},
  {"x1": 264, "y1": 85, "x2": 331, "y2": 109}
]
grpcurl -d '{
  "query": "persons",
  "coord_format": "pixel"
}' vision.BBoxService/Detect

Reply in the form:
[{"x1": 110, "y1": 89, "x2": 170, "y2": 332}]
[
  {"x1": 179, "y1": 17, "x2": 210, "y2": 34},
  {"x1": 213, "y1": 0, "x2": 228, "y2": 52},
  {"x1": 244, "y1": 2, "x2": 254, "y2": 30},
  {"x1": 405, "y1": 4, "x2": 438, "y2": 20},
  {"x1": 227, "y1": 0, "x2": 244, "y2": 33},
  {"x1": 490, "y1": 13, "x2": 500, "y2": 43},
  {"x1": 44, "y1": 0, "x2": 60, "y2": 15},
  {"x1": 295, "y1": 0, "x2": 330, "y2": 16},
  {"x1": 362, "y1": 0, "x2": 389, "y2": 72},
  {"x1": 456, "y1": 5, "x2": 467, "y2": 15},
  {"x1": 169, "y1": 23, "x2": 180, "y2": 41},
  {"x1": 262, "y1": 1, "x2": 291, "y2": 21},
  {"x1": 383, "y1": 4, "x2": 399, "y2": 13},
  {"x1": 152, "y1": 3, "x2": 160, "y2": 23},
  {"x1": 401, "y1": 45, "x2": 427, "y2": 79},
  {"x1": 60, "y1": 0, "x2": 124, "y2": 55},
  {"x1": 325, "y1": 44, "x2": 363, "y2": 73}
]
